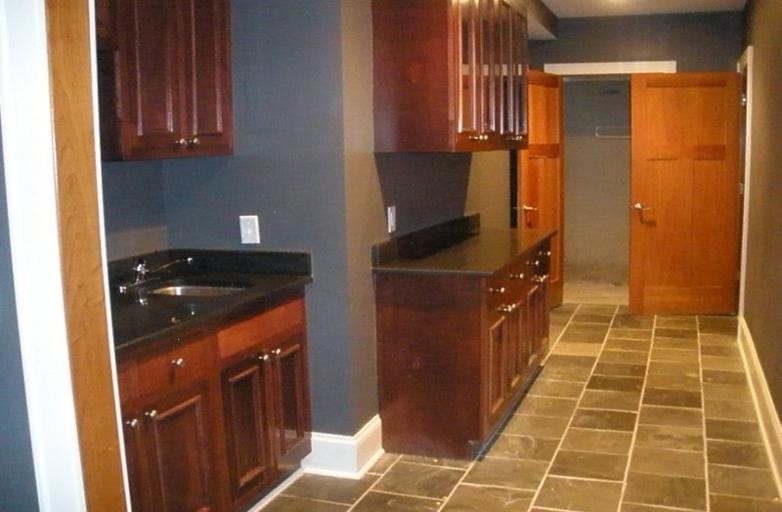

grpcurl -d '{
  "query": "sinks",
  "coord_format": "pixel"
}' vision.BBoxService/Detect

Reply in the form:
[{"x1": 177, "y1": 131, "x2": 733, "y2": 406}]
[{"x1": 147, "y1": 284, "x2": 246, "y2": 297}]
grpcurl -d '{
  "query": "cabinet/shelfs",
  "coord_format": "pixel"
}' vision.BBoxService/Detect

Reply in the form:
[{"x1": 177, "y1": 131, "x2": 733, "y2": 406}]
[
  {"x1": 373, "y1": 214, "x2": 559, "y2": 461},
  {"x1": 372, "y1": 0, "x2": 529, "y2": 155}
]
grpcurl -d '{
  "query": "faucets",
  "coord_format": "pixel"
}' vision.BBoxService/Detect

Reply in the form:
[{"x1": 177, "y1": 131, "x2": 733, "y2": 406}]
[{"x1": 131, "y1": 256, "x2": 194, "y2": 283}]
